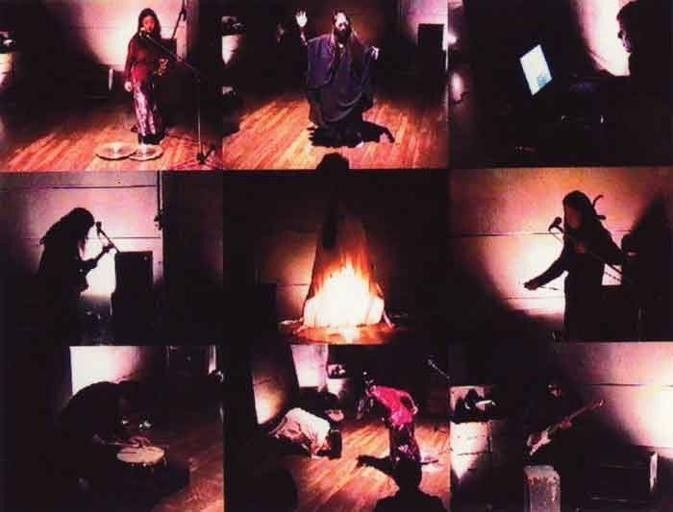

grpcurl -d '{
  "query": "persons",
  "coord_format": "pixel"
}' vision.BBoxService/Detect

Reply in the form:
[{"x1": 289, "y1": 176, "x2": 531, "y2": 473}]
[
  {"x1": 367, "y1": 459, "x2": 448, "y2": 512},
  {"x1": 267, "y1": 407, "x2": 342, "y2": 460},
  {"x1": 56, "y1": 380, "x2": 139, "y2": 443},
  {"x1": 524, "y1": 190, "x2": 624, "y2": 338},
  {"x1": 124, "y1": 8, "x2": 166, "y2": 144},
  {"x1": 567, "y1": 0, "x2": 673, "y2": 164},
  {"x1": 542, "y1": 377, "x2": 594, "y2": 474},
  {"x1": 350, "y1": 368, "x2": 419, "y2": 467},
  {"x1": 37, "y1": 208, "x2": 97, "y2": 297},
  {"x1": 294, "y1": 9, "x2": 384, "y2": 148}
]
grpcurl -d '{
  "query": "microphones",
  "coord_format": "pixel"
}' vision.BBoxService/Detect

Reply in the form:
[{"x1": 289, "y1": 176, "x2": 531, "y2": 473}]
[
  {"x1": 547, "y1": 215, "x2": 563, "y2": 234},
  {"x1": 95, "y1": 220, "x2": 102, "y2": 237},
  {"x1": 135, "y1": 26, "x2": 147, "y2": 41}
]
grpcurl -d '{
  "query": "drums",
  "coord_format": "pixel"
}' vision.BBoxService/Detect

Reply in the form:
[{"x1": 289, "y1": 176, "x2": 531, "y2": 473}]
[{"x1": 115, "y1": 443, "x2": 166, "y2": 470}]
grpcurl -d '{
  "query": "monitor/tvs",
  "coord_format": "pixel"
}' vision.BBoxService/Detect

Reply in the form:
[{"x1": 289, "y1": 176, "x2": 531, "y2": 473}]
[{"x1": 519, "y1": 41, "x2": 554, "y2": 96}]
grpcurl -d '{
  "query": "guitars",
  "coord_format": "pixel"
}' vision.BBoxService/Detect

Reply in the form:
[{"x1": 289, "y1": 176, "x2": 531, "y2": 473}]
[
  {"x1": 526, "y1": 397, "x2": 604, "y2": 455},
  {"x1": 69, "y1": 243, "x2": 114, "y2": 291}
]
